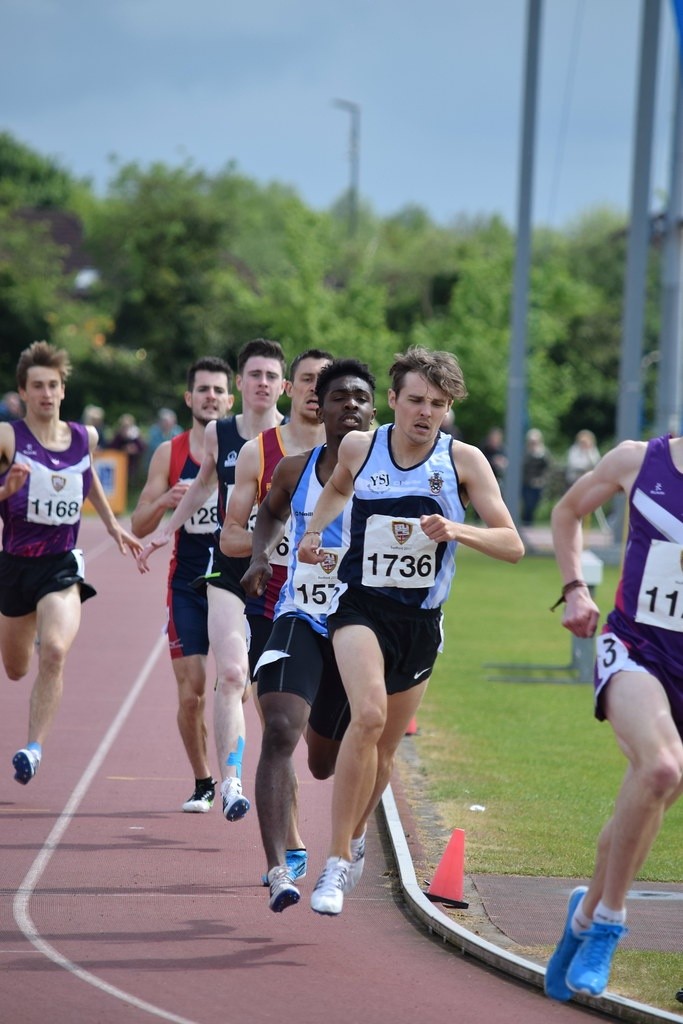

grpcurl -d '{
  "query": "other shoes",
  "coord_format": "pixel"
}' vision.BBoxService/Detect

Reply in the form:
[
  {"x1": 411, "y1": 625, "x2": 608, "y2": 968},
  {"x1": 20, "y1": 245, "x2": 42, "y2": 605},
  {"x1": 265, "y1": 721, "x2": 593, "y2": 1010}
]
[{"x1": 405, "y1": 716, "x2": 416, "y2": 736}]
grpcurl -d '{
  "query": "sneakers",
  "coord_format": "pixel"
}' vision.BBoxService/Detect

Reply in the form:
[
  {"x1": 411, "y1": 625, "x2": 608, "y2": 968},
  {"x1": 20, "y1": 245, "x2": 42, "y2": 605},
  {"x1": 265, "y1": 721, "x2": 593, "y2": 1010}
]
[
  {"x1": 268, "y1": 866, "x2": 300, "y2": 913},
  {"x1": 565, "y1": 923, "x2": 628, "y2": 998},
  {"x1": 182, "y1": 779, "x2": 218, "y2": 813},
  {"x1": 11, "y1": 749, "x2": 40, "y2": 785},
  {"x1": 263, "y1": 850, "x2": 307, "y2": 887},
  {"x1": 342, "y1": 822, "x2": 368, "y2": 896},
  {"x1": 222, "y1": 783, "x2": 250, "y2": 823},
  {"x1": 309, "y1": 856, "x2": 351, "y2": 915},
  {"x1": 544, "y1": 886, "x2": 590, "y2": 1002}
]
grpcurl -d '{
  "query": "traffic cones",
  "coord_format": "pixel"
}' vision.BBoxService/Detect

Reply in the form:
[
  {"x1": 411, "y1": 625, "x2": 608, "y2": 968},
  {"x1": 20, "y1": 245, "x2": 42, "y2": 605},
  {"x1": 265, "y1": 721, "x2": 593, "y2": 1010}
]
[
  {"x1": 422, "y1": 829, "x2": 469, "y2": 910},
  {"x1": 405, "y1": 717, "x2": 418, "y2": 736}
]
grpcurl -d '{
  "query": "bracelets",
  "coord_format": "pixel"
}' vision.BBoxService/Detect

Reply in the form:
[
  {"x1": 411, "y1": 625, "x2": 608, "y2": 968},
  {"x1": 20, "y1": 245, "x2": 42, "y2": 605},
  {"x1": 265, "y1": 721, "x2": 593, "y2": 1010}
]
[
  {"x1": 298, "y1": 532, "x2": 320, "y2": 545},
  {"x1": 550, "y1": 579, "x2": 588, "y2": 610}
]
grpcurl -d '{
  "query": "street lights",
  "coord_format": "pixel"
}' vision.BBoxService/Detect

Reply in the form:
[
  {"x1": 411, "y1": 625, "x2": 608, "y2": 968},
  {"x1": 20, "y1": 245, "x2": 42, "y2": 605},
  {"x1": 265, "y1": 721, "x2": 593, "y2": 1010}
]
[{"x1": 338, "y1": 99, "x2": 360, "y2": 234}]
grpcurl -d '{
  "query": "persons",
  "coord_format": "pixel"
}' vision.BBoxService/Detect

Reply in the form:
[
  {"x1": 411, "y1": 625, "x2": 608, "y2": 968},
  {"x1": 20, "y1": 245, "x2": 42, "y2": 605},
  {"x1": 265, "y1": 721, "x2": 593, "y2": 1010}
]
[
  {"x1": 482, "y1": 427, "x2": 510, "y2": 492},
  {"x1": 544, "y1": 435, "x2": 682, "y2": 1017},
  {"x1": 129, "y1": 356, "x2": 251, "y2": 814},
  {"x1": 239, "y1": 359, "x2": 377, "y2": 914},
  {"x1": 523, "y1": 428, "x2": 549, "y2": 526},
  {"x1": 146, "y1": 408, "x2": 183, "y2": 471},
  {"x1": 297, "y1": 348, "x2": 524, "y2": 915},
  {"x1": 218, "y1": 347, "x2": 336, "y2": 887},
  {"x1": 566, "y1": 429, "x2": 600, "y2": 529},
  {"x1": 438, "y1": 410, "x2": 460, "y2": 440},
  {"x1": 79, "y1": 407, "x2": 106, "y2": 450},
  {"x1": 0, "y1": 341, "x2": 142, "y2": 787},
  {"x1": 138, "y1": 339, "x2": 294, "y2": 822},
  {"x1": 112, "y1": 413, "x2": 145, "y2": 479},
  {"x1": 0, "y1": 390, "x2": 22, "y2": 421}
]
